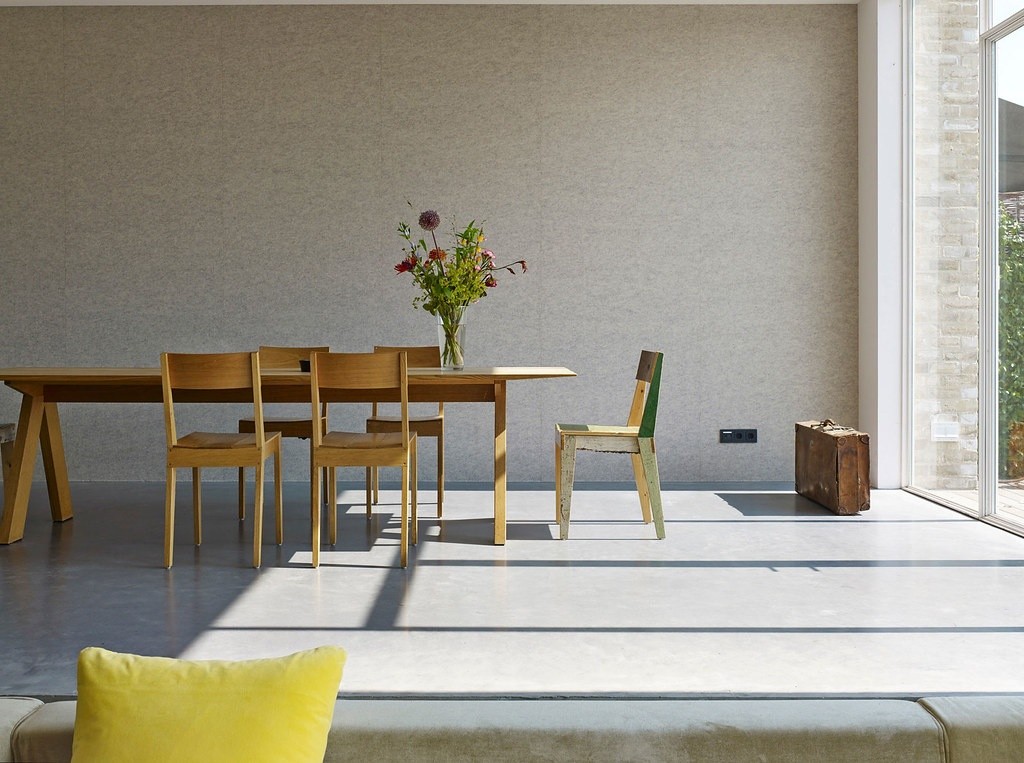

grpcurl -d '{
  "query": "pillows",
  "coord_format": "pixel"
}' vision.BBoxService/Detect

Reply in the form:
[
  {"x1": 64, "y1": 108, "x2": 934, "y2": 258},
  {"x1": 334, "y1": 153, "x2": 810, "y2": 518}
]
[{"x1": 66, "y1": 645, "x2": 348, "y2": 763}]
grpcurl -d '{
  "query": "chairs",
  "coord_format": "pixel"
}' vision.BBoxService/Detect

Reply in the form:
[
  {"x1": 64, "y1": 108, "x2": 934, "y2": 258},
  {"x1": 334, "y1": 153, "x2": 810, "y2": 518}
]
[
  {"x1": 365, "y1": 345, "x2": 445, "y2": 519},
  {"x1": 239, "y1": 346, "x2": 331, "y2": 520},
  {"x1": 159, "y1": 351, "x2": 284, "y2": 571},
  {"x1": 0, "y1": 423, "x2": 16, "y2": 496},
  {"x1": 554, "y1": 350, "x2": 664, "y2": 540},
  {"x1": 309, "y1": 351, "x2": 419, "y2": 568}
]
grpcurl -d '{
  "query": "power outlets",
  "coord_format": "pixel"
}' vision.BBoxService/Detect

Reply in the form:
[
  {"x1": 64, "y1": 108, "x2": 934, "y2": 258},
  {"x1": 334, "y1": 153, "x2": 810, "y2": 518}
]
[{"x1": 720, "y1": 429, "x2": 758, "y2": 443}]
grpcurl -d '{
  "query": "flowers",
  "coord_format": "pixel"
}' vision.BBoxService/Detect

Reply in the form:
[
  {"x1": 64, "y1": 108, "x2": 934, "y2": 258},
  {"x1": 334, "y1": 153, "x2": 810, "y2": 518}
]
[{"x1": 392, "y1": 192, "x2": 528, "y2": 306}]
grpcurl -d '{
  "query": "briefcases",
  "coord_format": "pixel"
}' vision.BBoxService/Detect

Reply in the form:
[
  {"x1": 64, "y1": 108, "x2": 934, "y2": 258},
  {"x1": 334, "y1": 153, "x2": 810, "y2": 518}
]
[{"x1": 792, "y1": 419, "x2": 870, "y2": 515}]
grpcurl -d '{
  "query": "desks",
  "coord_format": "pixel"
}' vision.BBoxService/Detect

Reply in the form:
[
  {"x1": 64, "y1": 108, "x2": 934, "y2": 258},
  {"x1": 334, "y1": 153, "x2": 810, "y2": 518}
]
[{"x1": 0, "y1": 366, "x2": 577, "y2": 546}]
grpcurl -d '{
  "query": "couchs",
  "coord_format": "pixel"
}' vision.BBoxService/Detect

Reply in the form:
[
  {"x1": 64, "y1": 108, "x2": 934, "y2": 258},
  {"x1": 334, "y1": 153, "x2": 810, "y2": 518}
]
[{"x1": 1, "y1": 696, "x2": 1024, "y2": 763}]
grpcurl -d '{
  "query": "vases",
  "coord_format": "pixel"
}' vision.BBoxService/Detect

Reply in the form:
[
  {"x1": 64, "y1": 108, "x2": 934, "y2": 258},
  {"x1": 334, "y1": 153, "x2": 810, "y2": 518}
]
[{"x1": 435, "y1": 307, "x2": 466, "y2": 371}]
